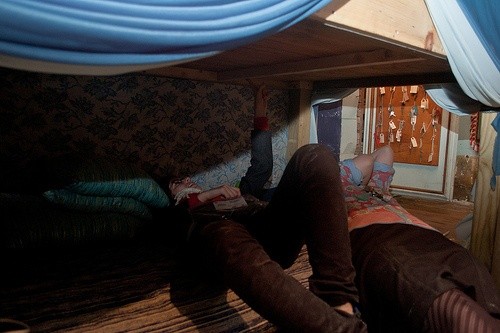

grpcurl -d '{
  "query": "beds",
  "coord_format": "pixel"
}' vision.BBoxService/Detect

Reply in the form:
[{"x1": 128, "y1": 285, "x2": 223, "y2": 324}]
[{"x1": 1, "y1": 235, "x2": 365, "y2": 333}]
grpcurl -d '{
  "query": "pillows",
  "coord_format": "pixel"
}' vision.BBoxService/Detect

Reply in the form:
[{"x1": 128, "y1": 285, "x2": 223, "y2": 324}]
[
  {"x1": 42, "y1": 184, "x2": 155, "y2": 221},
  {"x1": 6, "y1": 212, "x2": 146, "y2": 252},
  {"x1": 69, "y1": 158, "x2": 171, "y2": 207}
]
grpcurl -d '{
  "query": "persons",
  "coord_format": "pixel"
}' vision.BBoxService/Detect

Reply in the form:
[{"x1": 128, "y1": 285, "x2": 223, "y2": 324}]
[{"x1": 159, "y1": 80, "x2": 373, "y2": 333}]
